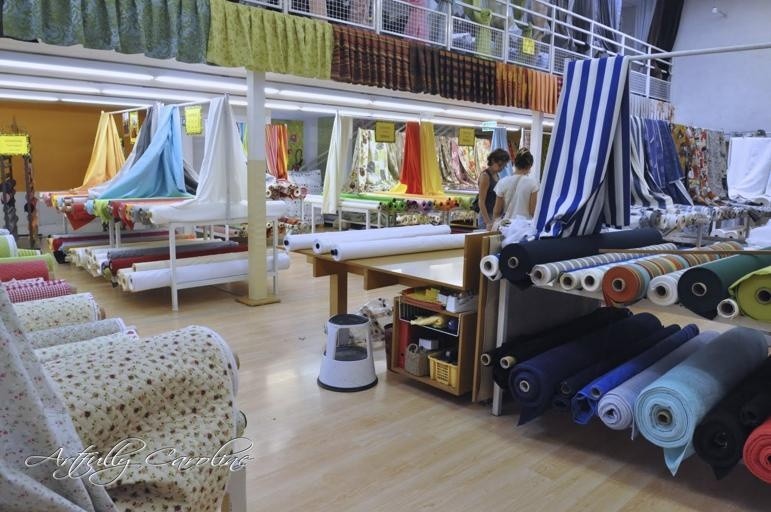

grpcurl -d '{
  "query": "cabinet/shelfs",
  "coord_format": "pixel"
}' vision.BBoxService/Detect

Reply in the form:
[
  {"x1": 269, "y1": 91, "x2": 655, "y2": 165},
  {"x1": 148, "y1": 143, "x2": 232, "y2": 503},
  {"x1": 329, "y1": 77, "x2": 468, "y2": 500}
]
[
  {"x1": 56, "y1": 205, "x2": 280, "y2": 311},
  {"x1": 390, "y1": 296, "x2": 477, "y2": 397},
  {"x1": 308, "y1": 203, "x2": 479, "y2": 235}
]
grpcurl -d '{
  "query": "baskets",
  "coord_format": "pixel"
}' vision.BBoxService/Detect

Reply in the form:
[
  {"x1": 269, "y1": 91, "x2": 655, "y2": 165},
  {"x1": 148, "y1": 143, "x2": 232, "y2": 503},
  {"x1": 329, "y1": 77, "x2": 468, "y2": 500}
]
[{"x1": 405, "y1": 344, "x2": 458, "y2": 388}]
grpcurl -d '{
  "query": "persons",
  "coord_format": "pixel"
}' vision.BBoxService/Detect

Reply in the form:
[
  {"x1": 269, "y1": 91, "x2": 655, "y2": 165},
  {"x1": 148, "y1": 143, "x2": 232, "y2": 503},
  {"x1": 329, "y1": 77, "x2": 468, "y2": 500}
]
[{"x1": 472, "y1": 147, "x2": 540, "y2": 229}]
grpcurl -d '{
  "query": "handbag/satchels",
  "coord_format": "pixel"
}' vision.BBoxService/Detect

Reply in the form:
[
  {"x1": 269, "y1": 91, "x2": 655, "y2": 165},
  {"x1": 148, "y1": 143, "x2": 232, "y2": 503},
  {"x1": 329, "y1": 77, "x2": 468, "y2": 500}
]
[
  {"x1": 491, "y1": 218, "x2": 502, "y2": 231},
  {"x1": 470, "y1": 198, "x2": 480, "y2": 213}
]
